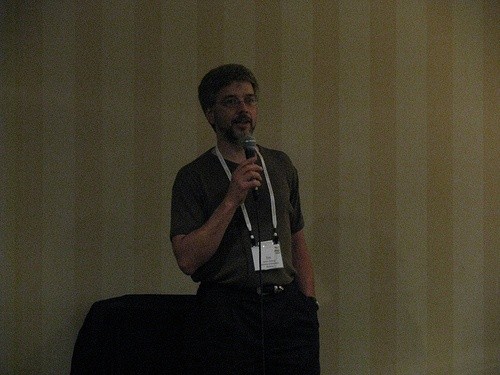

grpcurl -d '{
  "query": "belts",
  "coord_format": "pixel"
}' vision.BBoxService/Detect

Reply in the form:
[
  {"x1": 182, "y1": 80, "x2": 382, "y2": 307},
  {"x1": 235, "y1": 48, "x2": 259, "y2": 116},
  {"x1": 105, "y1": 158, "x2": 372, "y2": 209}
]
[{"x1": 200, "y1": 281, "x2": 296, "y2": 298}]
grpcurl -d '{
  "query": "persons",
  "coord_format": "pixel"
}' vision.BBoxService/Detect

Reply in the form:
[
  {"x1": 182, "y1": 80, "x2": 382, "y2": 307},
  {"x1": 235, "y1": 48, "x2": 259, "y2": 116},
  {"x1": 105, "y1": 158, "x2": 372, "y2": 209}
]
[{"x1": 169, "y1": 62, "x2": 321, "y2": 375}]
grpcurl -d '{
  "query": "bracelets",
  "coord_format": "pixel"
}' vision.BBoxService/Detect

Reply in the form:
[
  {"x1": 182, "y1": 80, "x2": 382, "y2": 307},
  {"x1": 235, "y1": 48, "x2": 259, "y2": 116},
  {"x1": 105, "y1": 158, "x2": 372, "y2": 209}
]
[{"x1": 308, "y1": 294, "x2": 320, "y2": 311}]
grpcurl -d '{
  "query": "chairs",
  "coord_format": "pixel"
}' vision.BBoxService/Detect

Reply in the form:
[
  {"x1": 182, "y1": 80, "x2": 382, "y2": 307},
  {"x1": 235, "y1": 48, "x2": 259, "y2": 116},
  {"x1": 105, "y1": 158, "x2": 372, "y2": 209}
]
[{"x1": 69, "y1": 294, "x2": 195, "y2": 375}]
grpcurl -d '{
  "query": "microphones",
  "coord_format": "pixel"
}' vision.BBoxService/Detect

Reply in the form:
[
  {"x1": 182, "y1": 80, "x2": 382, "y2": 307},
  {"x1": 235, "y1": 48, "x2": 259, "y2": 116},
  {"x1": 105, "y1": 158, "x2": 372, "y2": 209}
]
[{"x1": 243, "y1": 135, "x2": 259, "y2": 201}]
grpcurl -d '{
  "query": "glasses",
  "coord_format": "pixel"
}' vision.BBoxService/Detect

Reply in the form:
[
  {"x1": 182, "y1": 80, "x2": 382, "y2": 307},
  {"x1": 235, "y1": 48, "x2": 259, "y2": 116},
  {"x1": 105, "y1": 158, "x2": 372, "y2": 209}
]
[{"x1": 216, "y1": 95, "x2": 258, "y2": 109}]
